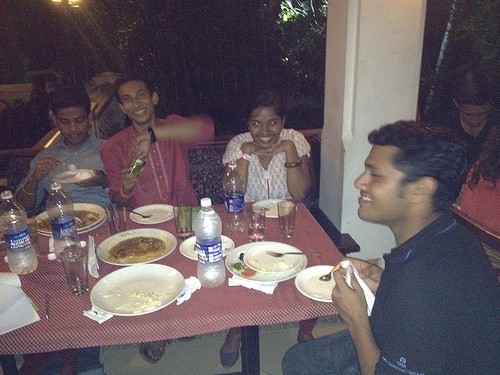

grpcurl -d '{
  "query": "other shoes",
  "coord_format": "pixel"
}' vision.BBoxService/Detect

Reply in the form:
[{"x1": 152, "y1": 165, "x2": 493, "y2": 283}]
[
  {"x1": 221, "y1": 336, "x2": 241, "y2": 368},
  {"x1": 296, "y1": 332, "x2": 315, "y2": 344}
]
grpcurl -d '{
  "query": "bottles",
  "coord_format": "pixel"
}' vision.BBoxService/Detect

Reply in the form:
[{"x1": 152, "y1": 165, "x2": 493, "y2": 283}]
[
  {"x1": 222, "y1": 159, "x2": 245, "y2": 233},
  {"x1": 0, "y1": 191, "x2": 38, "y2": 275},
  {"x1": 46, "y1": 181, "x2": 81, "y2": 261},
  {"x1": 191, "y1": 197, "x2": 226, "y2": 287}
]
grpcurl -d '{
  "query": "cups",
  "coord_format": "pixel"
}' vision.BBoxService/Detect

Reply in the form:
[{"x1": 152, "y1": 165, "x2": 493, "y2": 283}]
[
  {"x1": 173, "y1": 203, "x2": 192, "y2": 239},
  {"x1": 123, "y1": 153, "x2": 147, "y2": 180},
  {"x1": 61, "y1": 248, "x2": 88, "y2": 294},
  {"x1": 277, "y1": 200, "x2": 296, "y2": 238},
  {"x1": 106, "y1": 204, "x2": 126, "y2": 234},
  {"x1": 246, "y1": 206, "x2": 266, "y2": 243}
]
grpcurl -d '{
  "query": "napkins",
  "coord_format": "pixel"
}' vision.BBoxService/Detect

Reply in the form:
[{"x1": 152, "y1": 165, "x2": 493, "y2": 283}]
[
  {"x1": 339, "y1": 259, "x2": 376, "y2": 316},
  {"x1": 227, "y1": 276, "x2": 279, "y2": 296},
  {"x1": 176, "y1": 276, "x2": 202, "y2": 307},
  {"x1": 88, "y1": 235, "x2": 100, "y2": 278}
]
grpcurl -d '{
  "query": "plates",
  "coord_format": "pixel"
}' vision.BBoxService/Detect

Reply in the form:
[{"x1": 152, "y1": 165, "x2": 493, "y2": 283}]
[
  {"x1": 225, "y1": 241, "x2": 308, "y2": 283},
  {"x1": 295, "y1": 266, "x2": 338, "y2": 303},
  {"x1": 252, "y1": 199, "x2": 298, "y2": 219},
  {"x1": 0, "y1": 169, "x2": 106, "y2": 245},
  {"x1": 243, "y1": 243, "x2": 301, "y2": 272},
  {"x1": 179, "y1": 234, "x2": 235, "y2": 262},
  {"x1": 89, "y1": 263, "x2": 185, "y2": 317},
  {"x1": 129, "y1": 203, "x2": 173, "y2": 224},
  {"x1": 96, "y1": 229, "x2": 177, "y2": 266}
]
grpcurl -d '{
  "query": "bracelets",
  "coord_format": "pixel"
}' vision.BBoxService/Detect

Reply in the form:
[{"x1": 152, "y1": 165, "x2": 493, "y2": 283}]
[
  {"x1": 144, "y1": 126, "x2": 157, "y2": 142},
  {"x1": 21, "y1": 187, "x2": 36, "y2": 196},
  {"x1": 120, "y1": 187, "x2": 132, "y2": 199},
  {"x1": 285, "y1": 159, "x2": 303, "y2": 168}
]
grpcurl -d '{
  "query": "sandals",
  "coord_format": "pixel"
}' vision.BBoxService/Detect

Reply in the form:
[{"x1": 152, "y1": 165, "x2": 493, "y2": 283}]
[{"x1": 139, "y1": 342, "x2": 165, "y2": 364}]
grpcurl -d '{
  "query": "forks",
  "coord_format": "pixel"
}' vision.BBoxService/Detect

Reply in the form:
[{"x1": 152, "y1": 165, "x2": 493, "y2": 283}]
[
  {"x1": 266, "y1": 251, "x2": 320, "y2": 257},
  {"x1": 126, "y1": 208, "x2": 152, "y2": 219}
]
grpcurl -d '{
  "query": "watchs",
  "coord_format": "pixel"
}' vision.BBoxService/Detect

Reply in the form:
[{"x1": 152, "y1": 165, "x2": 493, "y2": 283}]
[
  {"x1": 101, "y1": 168, "x2": 111, "y2": 191},
  {"x1": 236, "y1": 150, "x2": 250, "y2": 161}
]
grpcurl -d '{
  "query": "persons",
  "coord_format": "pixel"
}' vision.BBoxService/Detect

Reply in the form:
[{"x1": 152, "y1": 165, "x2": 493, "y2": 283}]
[
  {"x1": 282, "y1": 118, "x2": 500, "y2": 375},
  {"x1": 11, "y1": 70, "x2": 325, "y2": 375},
  {"x1": 429, "y1": 68, "x2": 500, "y2": 236}
]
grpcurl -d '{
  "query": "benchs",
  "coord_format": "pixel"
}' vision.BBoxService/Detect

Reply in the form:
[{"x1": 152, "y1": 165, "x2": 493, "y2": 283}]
[{"x1": 6, "y1": 138, "x2": 321, "y2": 207}]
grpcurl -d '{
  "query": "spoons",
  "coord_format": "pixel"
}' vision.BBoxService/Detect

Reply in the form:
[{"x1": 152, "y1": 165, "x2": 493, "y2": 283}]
[
  {"x1": 320, "y1": 261, "x2": 340, "y2": 281},
  {"x1": 56, "y1": 160, "x2": 76, "y2": 171}
]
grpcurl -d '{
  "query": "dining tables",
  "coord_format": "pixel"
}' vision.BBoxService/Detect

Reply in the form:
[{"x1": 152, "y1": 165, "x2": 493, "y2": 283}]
[{"x1": 0, "y1": 201, "x2": 348, "y2": 375}]
